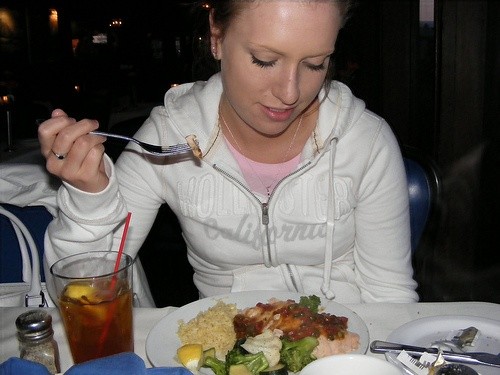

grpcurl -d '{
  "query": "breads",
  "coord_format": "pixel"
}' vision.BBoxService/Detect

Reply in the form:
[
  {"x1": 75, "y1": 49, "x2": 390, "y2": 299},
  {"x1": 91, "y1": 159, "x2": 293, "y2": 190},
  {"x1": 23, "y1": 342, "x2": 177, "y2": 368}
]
[{"x1": 177, "y1": 300, "x2": 237, "y2": 356}]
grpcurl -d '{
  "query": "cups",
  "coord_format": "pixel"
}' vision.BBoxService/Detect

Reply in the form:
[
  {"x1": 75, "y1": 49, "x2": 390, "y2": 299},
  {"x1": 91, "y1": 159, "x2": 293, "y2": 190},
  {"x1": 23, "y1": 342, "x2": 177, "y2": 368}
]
[{"x1": 50, "y1": 250, "x2": 134, "y2": 366}]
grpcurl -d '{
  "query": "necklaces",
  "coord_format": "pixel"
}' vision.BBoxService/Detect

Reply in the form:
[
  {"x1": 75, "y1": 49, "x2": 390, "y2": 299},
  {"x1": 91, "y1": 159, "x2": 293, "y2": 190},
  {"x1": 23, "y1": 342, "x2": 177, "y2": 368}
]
[{"x1": 218, "y1": 104, "x2": 303, "y2": 200}]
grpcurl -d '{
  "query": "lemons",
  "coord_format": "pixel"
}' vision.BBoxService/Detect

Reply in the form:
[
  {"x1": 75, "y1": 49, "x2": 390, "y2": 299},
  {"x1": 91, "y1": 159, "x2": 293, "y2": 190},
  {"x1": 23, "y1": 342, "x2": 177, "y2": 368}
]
[
  {"x1": 177, "y1": 344, "x2": 204, "y2": 371},
  {"x1": 65, "y1": 285, "x2": 107, "y2": 318}
]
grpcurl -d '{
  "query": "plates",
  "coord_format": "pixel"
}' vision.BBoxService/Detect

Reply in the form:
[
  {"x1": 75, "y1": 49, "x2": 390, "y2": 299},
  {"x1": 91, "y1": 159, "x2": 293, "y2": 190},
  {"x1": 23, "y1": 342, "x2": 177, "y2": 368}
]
[
  {"x1": 385, "y1": 314, "x2": 500, "y2": 375},
  {"x1": 145, "y1": 289, "x2": 370, "y2": 375},
  {"x1": 299, "y1": 354, "x2": 403, "y2": 375}
]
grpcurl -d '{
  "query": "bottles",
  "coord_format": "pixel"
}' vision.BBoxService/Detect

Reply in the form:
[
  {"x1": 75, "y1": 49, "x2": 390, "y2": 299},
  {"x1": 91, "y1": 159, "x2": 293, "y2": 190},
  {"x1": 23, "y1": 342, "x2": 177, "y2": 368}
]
[{"x1": 15, "y1": 309, "x2": 63, "y2": 374}]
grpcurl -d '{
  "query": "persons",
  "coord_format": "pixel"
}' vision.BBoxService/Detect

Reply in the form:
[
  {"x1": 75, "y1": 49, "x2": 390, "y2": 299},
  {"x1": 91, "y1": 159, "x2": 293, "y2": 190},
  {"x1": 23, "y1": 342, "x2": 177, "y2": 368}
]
[{"x1": 36, "y1": 0, "x2": 418, "y2": 304}]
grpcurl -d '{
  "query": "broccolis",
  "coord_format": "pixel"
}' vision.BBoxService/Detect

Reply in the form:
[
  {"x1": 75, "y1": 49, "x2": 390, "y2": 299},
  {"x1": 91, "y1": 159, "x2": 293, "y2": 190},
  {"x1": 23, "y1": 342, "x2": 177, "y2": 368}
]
[{"x1": 205, "y1": 295, "x2": 321, "y2": 375}]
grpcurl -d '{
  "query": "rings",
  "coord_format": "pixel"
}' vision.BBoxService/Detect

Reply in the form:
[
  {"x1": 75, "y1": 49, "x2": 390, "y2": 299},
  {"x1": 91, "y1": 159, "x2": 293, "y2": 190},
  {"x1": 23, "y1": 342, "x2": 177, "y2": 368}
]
[{"x1": 50, "y1": 149, "x2": 68, "y2": 159}]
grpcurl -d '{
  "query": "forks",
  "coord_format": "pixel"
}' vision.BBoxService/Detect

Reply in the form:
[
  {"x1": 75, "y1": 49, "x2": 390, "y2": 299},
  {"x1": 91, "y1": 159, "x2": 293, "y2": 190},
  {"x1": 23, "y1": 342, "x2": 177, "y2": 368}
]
[{"x1": 89, "y1": 131, "x2": 194, "y2": 157}]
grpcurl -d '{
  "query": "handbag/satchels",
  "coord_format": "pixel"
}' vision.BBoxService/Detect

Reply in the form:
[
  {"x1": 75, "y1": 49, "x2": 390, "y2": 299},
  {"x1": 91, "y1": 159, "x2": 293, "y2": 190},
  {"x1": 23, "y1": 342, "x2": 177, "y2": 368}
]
[{"x1": 0, "y1": 205, "x2": 140, "y2": 308}]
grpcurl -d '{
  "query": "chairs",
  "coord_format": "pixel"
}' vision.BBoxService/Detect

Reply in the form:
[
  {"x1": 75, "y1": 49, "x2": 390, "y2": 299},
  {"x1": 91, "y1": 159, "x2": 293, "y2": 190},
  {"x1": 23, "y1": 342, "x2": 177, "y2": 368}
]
[{"x1": 401, "y1": 143, "x2": 444, "y2": 275}]
